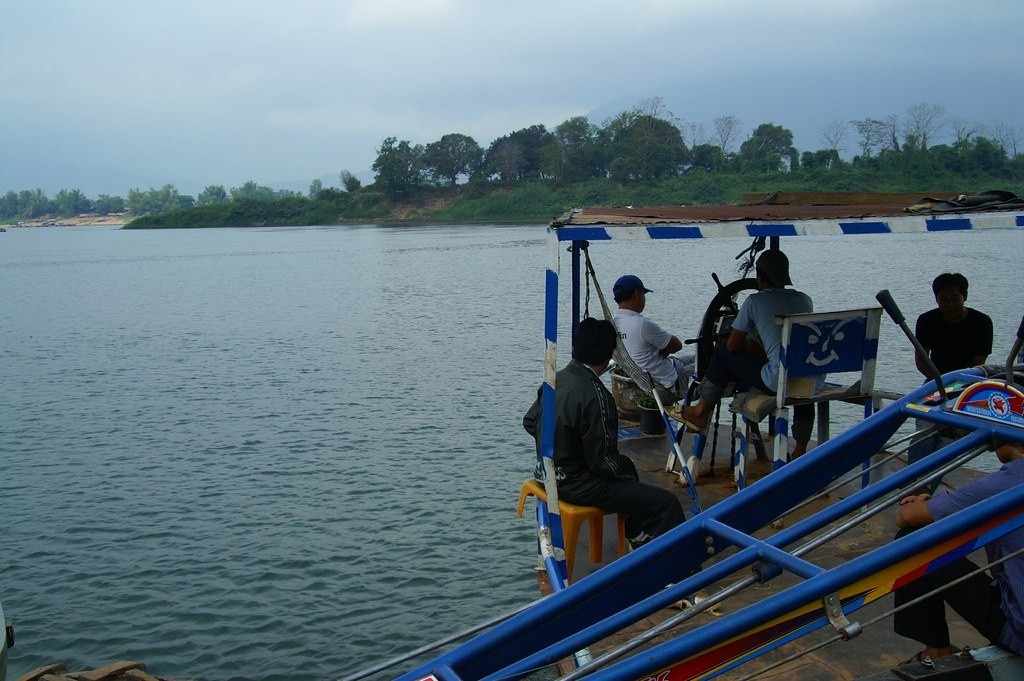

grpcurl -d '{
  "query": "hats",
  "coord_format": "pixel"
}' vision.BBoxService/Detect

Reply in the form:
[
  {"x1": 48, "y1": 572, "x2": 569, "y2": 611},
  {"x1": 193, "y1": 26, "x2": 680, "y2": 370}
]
[
  {"x1": 613, "y1": 275, "x2": 654, "y2": 296},
  {"x1": 756, "y1": 249, "x2": 793, "y2": 286}
]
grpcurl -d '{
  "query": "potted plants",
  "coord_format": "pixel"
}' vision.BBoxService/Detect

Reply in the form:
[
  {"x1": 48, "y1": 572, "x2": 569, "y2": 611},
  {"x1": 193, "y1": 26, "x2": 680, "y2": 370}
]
[{"x1": 632, "y1": 394, "x2": 667, "y2": 435}]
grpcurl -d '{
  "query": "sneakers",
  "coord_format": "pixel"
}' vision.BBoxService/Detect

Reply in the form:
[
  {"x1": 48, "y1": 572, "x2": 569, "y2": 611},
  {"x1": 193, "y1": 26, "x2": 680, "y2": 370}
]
[
  {"x1": 663, "y1": 582, "x2": 721, "y2": 612},
  {"x1": 628, "y1": 531, "x2": 656, "y2": 552}
]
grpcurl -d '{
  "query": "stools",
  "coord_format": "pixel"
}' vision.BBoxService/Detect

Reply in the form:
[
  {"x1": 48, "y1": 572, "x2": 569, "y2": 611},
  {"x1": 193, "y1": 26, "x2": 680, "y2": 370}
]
[{"x1": 518, "y1": 479, "x2": 626, "y2": 586}]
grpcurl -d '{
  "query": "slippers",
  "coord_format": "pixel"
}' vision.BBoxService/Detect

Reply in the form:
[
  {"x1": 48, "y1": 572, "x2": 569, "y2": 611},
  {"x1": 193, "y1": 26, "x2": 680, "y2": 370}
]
[{"x1": 664, "y1": 404, "x2": 706, "y2": 431}]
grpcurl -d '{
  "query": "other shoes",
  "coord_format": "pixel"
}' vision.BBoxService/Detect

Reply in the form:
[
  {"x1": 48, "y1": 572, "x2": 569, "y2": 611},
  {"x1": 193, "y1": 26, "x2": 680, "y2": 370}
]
[{"x1": 897, "y1": 650, "x2": 923, "y2": 666}]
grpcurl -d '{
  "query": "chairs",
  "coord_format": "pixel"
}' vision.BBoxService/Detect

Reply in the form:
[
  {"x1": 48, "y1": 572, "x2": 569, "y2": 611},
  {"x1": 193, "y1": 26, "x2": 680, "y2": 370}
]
[{"x1": 728, "y1": 306, "x2": 884, "y2": 492}]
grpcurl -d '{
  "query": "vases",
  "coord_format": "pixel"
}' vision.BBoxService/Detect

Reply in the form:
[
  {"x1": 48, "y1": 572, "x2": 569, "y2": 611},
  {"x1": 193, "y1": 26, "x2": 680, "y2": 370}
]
[{"x1": 610, "y1": 367, "x2": 646, "y2": 422}]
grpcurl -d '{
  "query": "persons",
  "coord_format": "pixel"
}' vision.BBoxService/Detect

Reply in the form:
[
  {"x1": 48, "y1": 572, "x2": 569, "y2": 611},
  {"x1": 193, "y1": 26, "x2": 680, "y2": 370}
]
[
  {"x1": 900, "y1": 272, "x2": 994, "y2": 506},
  {"x1": 613, "y1": 273, "x2": 696, "y2": 401},
  {"x1": 665, "y1": 249, "x2": 826, "y2": 461},
  {"x1": 893, "y1": 440, "x2": 1024, "y2": 666},
  {"x1": 523, "y1": 316, "x2": 722, "y2": 612}
]
